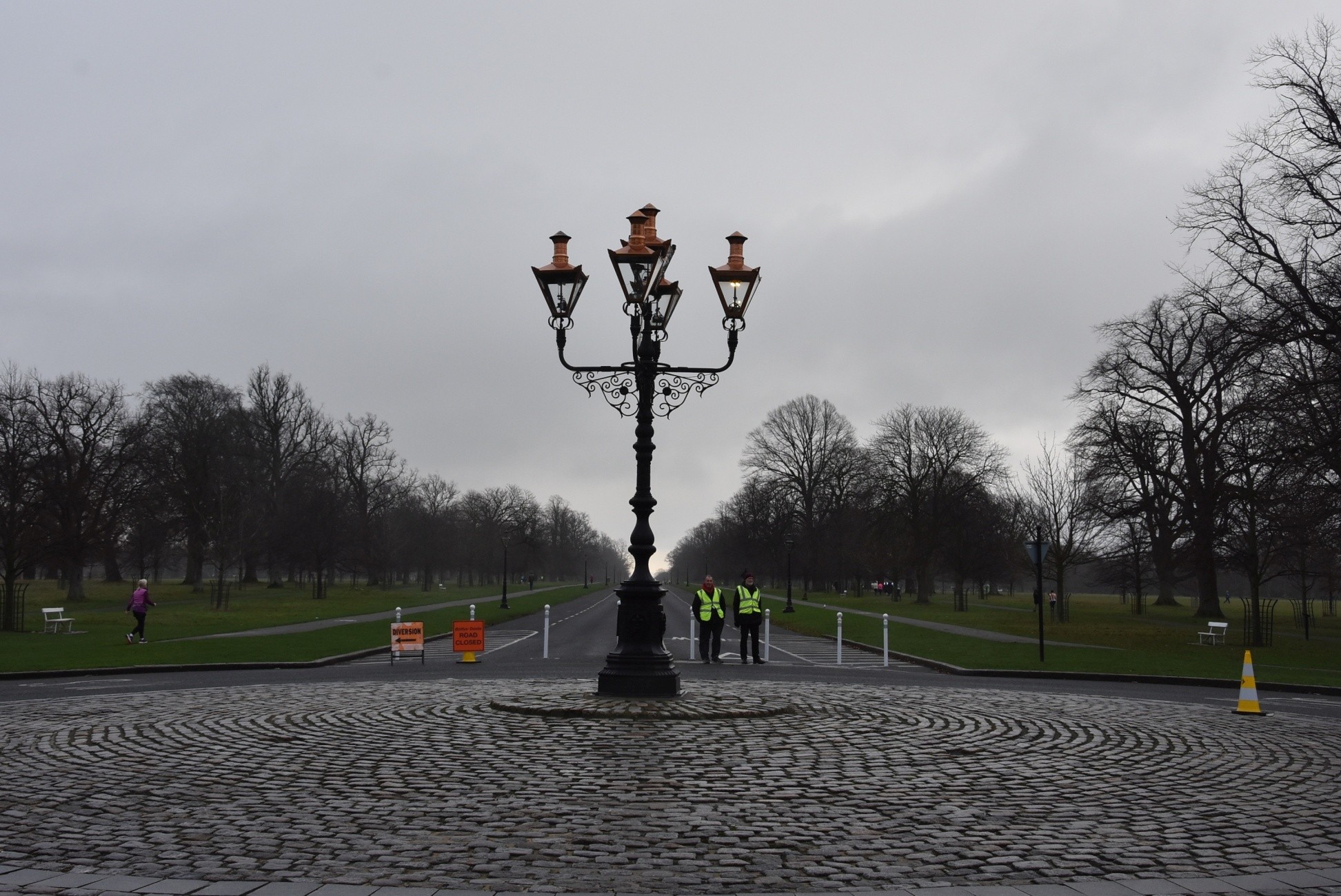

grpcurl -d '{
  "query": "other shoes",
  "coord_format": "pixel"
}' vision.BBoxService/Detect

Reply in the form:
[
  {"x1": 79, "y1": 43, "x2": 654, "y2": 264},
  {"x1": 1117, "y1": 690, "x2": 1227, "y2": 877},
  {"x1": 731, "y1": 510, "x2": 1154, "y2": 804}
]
[
  {"x1": 704, "y1": 659, "x2": 710, "y2": 665},
  {"x1": 753, "y1": 656, "x2": 766, "y2": 664},
  {"x1": 741, "y1": 659, "x2": 748, "y2": 664},
  {"x1": 713, "y1": 657, "x2": 723, "y2": 663}
]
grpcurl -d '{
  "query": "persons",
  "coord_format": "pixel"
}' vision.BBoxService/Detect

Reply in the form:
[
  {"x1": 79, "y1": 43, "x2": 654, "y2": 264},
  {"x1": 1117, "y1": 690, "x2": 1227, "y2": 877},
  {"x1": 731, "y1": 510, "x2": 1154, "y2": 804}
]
[
  {"x1": 873, "y1": 580, "x2": 883, "y2": 595},
  {"x1": 528, "y1": 571, "x2": 535, "y2": 590},
  {"x1": 1033, "y1": 589, "x2": 1039, "y2": 611},
  {"x1": 126, "y1": 578, "x2": 157, "y2": 645},
  {"x1": 1048, "y1": 590, "x2": 1056, "y2": 612},
  {"x1": 590, "y1": 574, "x2": 594, "y2": 584},
  {"x1": 733, "y1": 572, "x2": 765, "y2": 664},
  {"x1": 693, "y1": 574, "x2": 726, "y2": 664},
  {"x1": 1224, "y1": 590, "x2": 1230, "y2": 604}
]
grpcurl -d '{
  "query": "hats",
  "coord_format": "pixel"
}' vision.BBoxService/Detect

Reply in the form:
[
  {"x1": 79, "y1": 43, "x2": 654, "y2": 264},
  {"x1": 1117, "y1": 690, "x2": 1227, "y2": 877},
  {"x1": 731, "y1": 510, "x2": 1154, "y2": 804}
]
[{"x1": 743, "y1": 573, "x2": 755, "y2": 580}]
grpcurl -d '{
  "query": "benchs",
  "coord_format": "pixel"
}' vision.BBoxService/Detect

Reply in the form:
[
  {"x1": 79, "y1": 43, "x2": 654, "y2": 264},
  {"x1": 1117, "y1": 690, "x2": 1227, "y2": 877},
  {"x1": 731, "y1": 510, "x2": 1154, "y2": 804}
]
[
  {"x1": 42, "y1": 608, "x2": 74, "y2": 634},
  {"x1": 1197, "y1": 622, "x2": 1228, "y2": 647},
  {"x1": 439, "y1": 584, "x2": 446, "y2": 591},
  {"x1": 840, "y1": 591, "x2": 847, "y2": 598}
]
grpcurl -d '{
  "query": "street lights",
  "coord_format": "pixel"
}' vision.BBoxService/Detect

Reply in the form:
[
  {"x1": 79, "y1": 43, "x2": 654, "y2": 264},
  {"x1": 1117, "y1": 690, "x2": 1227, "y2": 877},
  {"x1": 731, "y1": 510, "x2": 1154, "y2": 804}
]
[
  {"x1": 531, "y1": 202, "x2": 763, "y2": 697},
  {"x1": 705, "y1": 555, "x2": 709, "y2": 575},
  {"x1": 583, "y1": 554, "x2": 589, "y2": 589},
  {"x1": 604, "y1": 561, "x2": 608, "y2": 586},
  {"x1": 498, "y1": 530, "x2": 513, "y2": 609},
  {"x1": 685, "y1": 563, "x2": 690, "y2": 587},
  {"x1": 676, "y1": 567, "x2": 680, "y2": 585},
  {"x1": 781, "y1": 533, "x2": 796, "y2": 613},
  {"x1": 613, "y1": 565, "x2": 616, "y2": 584}
]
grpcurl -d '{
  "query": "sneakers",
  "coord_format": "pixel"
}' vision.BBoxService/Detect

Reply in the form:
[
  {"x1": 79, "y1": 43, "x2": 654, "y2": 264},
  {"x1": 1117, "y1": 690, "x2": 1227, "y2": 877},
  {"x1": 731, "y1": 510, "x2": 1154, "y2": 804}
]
[
  {"x1": 125, "y1": 633, "x2": 133, "y2": 643},
  {"x1": 139, "y1": 639, "x2": 149, "y2": 644}
]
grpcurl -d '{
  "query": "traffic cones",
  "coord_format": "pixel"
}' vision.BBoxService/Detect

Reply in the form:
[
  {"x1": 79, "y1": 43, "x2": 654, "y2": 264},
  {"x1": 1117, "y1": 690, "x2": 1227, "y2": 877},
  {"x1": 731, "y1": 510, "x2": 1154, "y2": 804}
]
[{"x1": 1231, "y1": 650, "x2": 1268, "y2": 716}]
